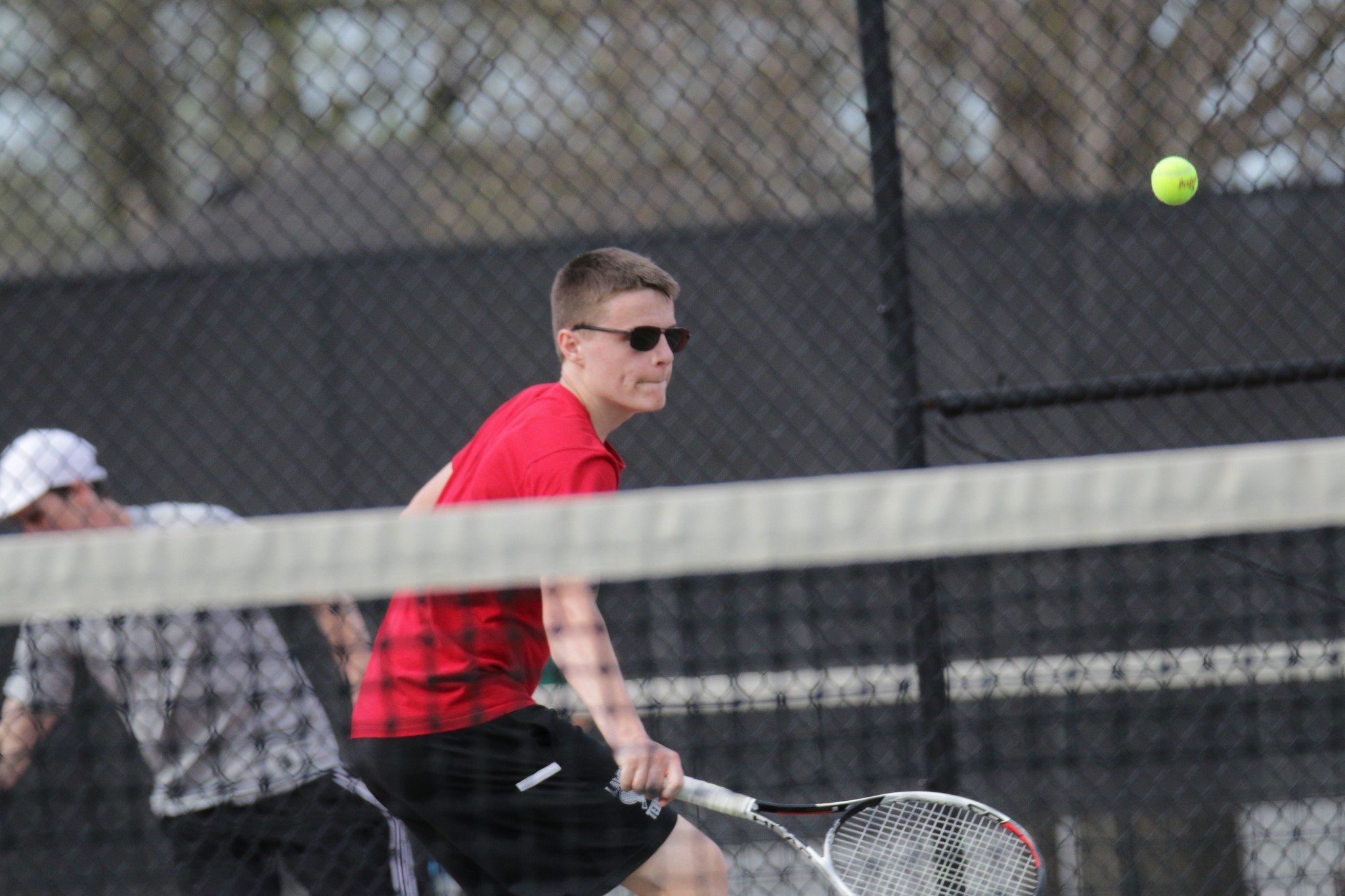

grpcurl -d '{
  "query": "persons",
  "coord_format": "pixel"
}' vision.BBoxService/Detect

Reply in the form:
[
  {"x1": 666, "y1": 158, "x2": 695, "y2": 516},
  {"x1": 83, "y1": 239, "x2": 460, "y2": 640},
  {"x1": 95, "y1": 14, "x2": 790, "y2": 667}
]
[{"x1": 351, "y1": 252, "x2": 729, "y2": 896}]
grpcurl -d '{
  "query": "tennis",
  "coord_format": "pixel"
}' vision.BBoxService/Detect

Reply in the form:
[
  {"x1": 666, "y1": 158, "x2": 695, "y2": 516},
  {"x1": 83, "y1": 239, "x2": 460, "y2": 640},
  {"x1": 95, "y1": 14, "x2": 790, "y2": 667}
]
[{"x1": 1150, "y1": 155, "x2": 1198, "y2": 205}]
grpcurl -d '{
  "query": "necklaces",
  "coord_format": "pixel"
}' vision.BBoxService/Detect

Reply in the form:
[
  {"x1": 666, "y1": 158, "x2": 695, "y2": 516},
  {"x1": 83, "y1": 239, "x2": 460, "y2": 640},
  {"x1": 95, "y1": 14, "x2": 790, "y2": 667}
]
[{"x1": 0, "y1": 432, "x2": 437, "y2": 896}]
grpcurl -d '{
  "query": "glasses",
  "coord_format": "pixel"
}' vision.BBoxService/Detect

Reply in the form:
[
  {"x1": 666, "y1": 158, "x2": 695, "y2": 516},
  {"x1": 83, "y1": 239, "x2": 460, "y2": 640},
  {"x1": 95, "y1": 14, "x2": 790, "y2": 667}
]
[{"x1": 572, "y1": 324, "x2": 690, "y2": 353}]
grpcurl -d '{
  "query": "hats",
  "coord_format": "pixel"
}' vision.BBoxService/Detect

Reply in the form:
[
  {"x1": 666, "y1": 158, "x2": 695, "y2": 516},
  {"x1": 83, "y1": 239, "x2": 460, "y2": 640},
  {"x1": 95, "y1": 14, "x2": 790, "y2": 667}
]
[{"x1": 1, "y1": 430, "x2": 111, "y2": 522}]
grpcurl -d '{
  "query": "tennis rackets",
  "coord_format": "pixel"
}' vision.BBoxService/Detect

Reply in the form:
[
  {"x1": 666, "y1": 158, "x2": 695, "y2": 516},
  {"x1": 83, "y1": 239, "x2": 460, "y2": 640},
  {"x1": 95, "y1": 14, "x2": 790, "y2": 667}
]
[{"x1": 615, "y1": 765, "x2": 1043, "y2": 895}]
